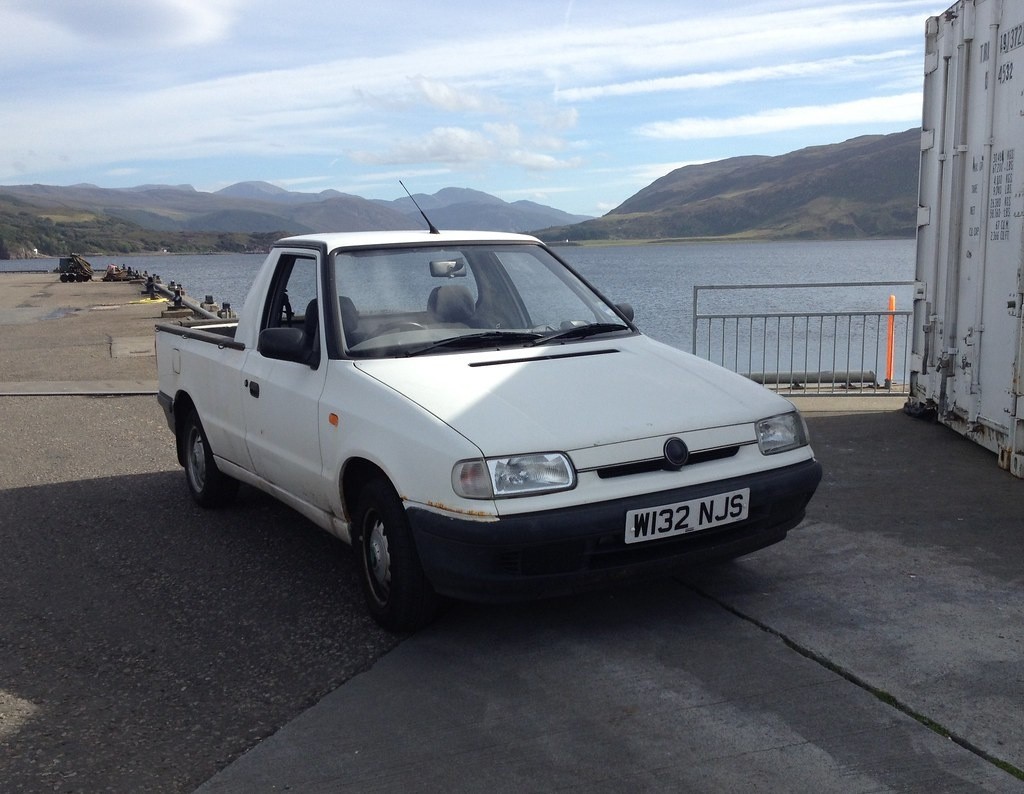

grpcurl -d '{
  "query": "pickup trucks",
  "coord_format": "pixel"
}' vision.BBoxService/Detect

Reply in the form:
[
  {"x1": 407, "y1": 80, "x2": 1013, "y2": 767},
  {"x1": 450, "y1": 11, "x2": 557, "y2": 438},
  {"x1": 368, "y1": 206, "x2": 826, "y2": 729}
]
[{"x1": 155, "y1": 229, "x2": 822, "y2": 637}]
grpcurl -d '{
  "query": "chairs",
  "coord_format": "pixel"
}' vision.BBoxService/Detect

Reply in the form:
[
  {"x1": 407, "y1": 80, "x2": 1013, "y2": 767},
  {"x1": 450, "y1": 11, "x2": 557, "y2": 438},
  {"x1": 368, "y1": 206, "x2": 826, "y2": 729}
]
[
  {"x1": 427, "y1": 285, "x2": 488, "y2": 328},
  {"x1": 304, "y1": 296, "x2": 374, "y2": 349}
]
